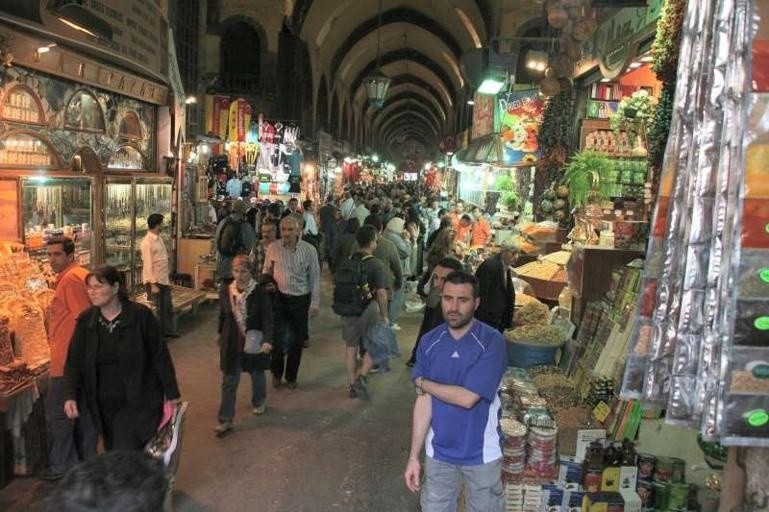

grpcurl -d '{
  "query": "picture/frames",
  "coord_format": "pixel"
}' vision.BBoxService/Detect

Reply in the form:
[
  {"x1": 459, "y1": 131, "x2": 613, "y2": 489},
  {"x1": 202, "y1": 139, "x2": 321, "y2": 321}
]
[{"x1": 194, "y1": 264, "x2": 221, "y2": 300}]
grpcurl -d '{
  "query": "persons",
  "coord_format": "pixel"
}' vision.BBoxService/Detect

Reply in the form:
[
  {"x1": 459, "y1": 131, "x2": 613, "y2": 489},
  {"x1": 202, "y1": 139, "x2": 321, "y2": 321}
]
[
  {"x1": 139, "y1": 214, "x2": 181, "y2": 337},
  {"x1": 405, "y1": 272, "x2": 510, "y2": 512},
  {"x1": 213, "y1": 180, "x2": 520, "y2": 432},
  {"x1": 47, "y1": 450, "x2": 176, "y2": 512},
  {"x1": 38, "y1": 237, "x2": 98, "y2": 480},
  {"x1": 62, "y1": 265, "x2": 181, "y2": 451}
]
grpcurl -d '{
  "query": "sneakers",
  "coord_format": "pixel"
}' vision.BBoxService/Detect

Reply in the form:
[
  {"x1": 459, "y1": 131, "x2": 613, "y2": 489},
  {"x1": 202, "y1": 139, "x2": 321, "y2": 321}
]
[
  {"x1": 354, "y1": 375, "x2": 370, "y2": 401},
  {"x1": 346, "y1": 383, "x2": 357, "y2": 398},
  {"x1": 213, "y1": 422, "x2": 233, "y2": 434},
  {"x1": 406, "y1": 359, "x2": 415, "y2": 367},
  {"x1": 286, "y1": 376, "x2": 297, "y2": 390},
  {"x1": 252, "y1": 398, "x2": 267, "y2": 414},
  {"x1": 159, "y1": 327, "x2": 183, "y2": 345},
  {"x1": 272, "y1": 375, "x2": 281, "y2": 388},
  {"x1": 390, "y1": 322, "x2": 402, "y2": 332},
  {"x1": 36, "y1": 465, "x2": 64, "y2": 482},
  {"x1": 355, "y1": 344, "x2": 382, "y2": 374}
]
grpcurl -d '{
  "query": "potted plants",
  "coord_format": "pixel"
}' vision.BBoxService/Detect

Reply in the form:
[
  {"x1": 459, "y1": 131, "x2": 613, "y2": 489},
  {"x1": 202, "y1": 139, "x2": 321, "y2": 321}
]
[{"x1": 565, "y1": 148, "x2": 610, "y2": 246}]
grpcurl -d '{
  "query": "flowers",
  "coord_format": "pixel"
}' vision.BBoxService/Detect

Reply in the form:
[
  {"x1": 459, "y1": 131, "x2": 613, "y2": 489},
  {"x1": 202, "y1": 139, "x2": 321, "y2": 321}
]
[{"x1": 609, "y1": 87, "x2": 656, "y2": 150}]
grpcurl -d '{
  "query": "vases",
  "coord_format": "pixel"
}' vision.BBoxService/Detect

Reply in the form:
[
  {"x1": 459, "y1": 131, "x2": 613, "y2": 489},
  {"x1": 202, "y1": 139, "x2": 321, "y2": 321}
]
[{"x1": 622, "y1": 108, "x2": 640, "y2": 118}]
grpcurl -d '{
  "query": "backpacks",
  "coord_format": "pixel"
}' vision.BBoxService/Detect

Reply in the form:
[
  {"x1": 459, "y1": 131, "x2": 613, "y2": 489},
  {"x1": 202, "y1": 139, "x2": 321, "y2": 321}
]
[
  {"x1": 217, "y1": 215, "x2": 248, "y2": 258},
  {"x1": 332, "y1": 251, "x2": 376, "y2": 318}
]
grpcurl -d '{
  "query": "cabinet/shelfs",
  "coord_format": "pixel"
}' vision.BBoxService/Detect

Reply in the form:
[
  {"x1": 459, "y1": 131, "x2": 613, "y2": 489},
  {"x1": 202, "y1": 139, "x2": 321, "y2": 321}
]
[{"x1": 578, "y1": 117, "x2": 652, "y2": 220}]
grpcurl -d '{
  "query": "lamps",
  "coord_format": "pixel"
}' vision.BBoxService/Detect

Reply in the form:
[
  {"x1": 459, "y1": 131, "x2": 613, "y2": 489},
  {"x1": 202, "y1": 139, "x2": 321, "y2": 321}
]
[
  {"x1": 365, "y1": 0, "x2": 392, "y2": 112},
  {"x1": 45, "y1": 0, "x2": 113, "y2": 43},
  {"x1": 525, "y1": 48, "x2": 550, "y2": 73}
]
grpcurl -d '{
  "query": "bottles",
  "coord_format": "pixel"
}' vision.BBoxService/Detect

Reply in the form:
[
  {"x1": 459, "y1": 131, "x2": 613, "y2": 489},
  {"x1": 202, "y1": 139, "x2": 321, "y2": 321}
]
[
  {"x1": 23, "y1": 222, "x2": 91, "y2": 246},
  {"x1": 578, "y1": 437, "x2": 637, "y2": 489},
  {"x1": 77, "y1": 249, "x2": 91, "y2": 265}
]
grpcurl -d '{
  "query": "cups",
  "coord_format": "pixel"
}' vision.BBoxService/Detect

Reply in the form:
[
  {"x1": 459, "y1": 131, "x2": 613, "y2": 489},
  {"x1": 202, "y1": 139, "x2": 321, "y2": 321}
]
[{"x1": 599, "y1": 230, "x2": 614, "y2": 249}]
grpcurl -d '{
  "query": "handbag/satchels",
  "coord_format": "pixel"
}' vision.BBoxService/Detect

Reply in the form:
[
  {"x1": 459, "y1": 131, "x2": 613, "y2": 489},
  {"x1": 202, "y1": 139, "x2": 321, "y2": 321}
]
[
  {"x1": 242, "y1": 328, "x2": 264, "y2": 355},
  {"x1": 423, "y1": 267, "x2": 437, "y2": 295},
  {"x1": 416, "y1": 269, "x2": 430, "y2": 298},
  {"x1": 143, "y1": 400, "x2": 190, "y2": 477}
]
